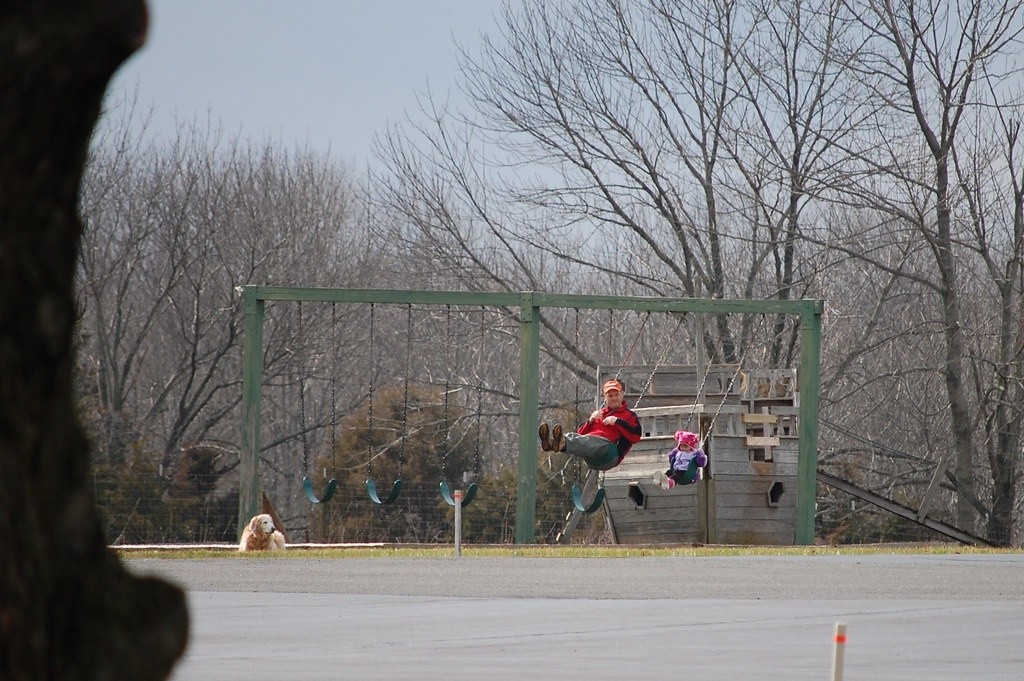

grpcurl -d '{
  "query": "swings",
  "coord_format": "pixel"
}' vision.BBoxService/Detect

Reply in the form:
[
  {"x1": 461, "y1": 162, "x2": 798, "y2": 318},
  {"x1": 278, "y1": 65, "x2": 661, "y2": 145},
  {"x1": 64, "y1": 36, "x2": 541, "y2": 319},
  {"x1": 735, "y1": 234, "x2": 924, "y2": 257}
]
[
  {"x1": 582, "y1": 312, "x2": 687, "y2": 468},
  {"x1": 571, "y1": 309, "x2": 614, "y2": 513},
  {"x1": 297, "y1": 302, "x2": 337, "y2": 504},
  {"x1": 365, "y1": 302, "x2": 412, "y2": 506},
  {"x1": 440, "y1": 304, "x2": 485, "y2": 507},
  {"x1": 669, "y1": 313, "x2": 766, "y2": 485}
]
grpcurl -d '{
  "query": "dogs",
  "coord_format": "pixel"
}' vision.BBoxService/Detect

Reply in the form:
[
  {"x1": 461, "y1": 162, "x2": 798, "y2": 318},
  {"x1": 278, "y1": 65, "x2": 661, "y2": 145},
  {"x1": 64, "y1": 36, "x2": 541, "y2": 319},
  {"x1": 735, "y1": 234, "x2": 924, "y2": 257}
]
[{"x1": 238, "y1": 513, "x2": 285, "y2": 554}]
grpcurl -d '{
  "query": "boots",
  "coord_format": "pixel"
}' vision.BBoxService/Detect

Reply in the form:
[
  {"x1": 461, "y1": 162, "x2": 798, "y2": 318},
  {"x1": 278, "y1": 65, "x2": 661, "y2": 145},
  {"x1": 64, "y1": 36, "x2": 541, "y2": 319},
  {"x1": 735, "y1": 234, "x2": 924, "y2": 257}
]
[
  {"x1": 661, "y1": 475, "x2": 675, "y2": 491},
  {"x1": 652, "y1": 470, "x2": 668, "y2": 486}
]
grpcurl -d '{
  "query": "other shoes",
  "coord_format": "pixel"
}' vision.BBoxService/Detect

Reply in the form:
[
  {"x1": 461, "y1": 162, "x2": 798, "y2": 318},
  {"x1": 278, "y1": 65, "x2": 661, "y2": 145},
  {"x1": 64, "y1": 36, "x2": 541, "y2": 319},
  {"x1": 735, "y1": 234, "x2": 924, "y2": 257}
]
[
  {"x1": 538, "y1": 422, "x2": 554, "y2": 452},
  {"x1": 551, "y1": 423, "x2": 566, "y2": 452}
]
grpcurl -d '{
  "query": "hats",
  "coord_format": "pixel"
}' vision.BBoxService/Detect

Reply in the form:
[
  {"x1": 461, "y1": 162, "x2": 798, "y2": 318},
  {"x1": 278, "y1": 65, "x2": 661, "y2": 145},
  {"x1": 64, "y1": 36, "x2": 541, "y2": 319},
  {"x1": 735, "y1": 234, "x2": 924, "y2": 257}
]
[
  {"x1": 604, "y1": 381, "x2": 622, "y2": 393},
  {"x1": 674, "y1": 430, "x2": 699, "y2": 450}
]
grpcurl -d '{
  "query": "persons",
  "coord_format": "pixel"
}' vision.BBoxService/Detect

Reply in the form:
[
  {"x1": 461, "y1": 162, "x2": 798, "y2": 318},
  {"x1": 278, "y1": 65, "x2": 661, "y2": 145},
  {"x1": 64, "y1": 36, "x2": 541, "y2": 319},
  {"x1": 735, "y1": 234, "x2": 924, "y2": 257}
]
[
  {"x1": 538, "y1": 379, "x2": 642, "y2": 470},
  {"x1": 0, "y1": 0, "x2": 196, "y2": 681},
  {"x1": 653, "y1": 429, "x2": 708, "y2": 490}
]
